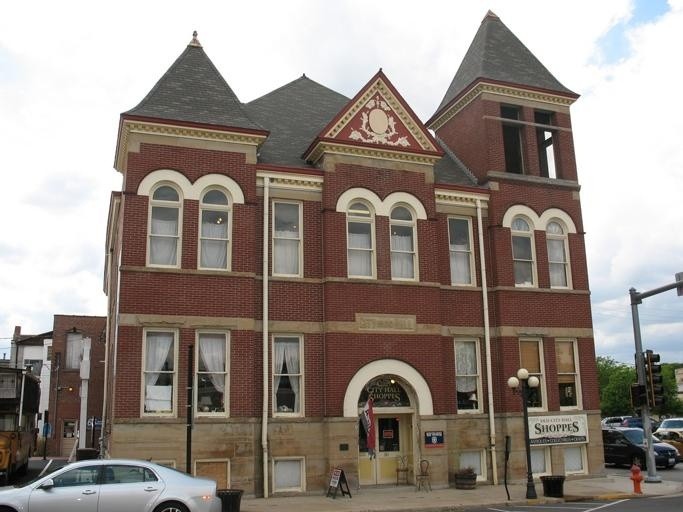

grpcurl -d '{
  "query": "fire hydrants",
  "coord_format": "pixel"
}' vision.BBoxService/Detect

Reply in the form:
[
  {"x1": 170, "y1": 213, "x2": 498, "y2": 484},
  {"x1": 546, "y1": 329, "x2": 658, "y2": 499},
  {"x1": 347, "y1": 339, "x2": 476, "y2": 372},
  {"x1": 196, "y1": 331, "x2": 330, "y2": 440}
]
[{"x1": 629, "y1": 462, "x2": 643, "y2": 494}]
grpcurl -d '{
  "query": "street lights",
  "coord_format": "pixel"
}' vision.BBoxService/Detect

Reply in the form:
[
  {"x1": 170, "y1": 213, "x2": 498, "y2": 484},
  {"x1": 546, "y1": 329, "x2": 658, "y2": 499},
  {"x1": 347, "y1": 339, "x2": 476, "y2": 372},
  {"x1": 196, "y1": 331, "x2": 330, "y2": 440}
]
[{"x1": 506, "y1": 367, "x2": 540, "y2": 500}]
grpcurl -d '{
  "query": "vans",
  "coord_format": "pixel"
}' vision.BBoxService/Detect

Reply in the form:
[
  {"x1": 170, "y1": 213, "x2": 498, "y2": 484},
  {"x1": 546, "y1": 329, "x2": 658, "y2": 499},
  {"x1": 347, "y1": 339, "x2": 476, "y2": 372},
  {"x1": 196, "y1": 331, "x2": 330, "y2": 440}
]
[{"x1": 653, "y1": 418, "x2": 682, "y2": 432}]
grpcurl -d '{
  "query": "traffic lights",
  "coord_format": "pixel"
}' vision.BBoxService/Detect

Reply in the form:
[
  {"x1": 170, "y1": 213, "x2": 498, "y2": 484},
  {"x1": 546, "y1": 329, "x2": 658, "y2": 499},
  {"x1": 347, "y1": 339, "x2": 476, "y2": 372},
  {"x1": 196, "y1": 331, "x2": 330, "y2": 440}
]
[
  {"x1": 649, "y1": 352, "x2": 665, "y2": 405},
  {"x1": 633, "y1": 383, "x2": 647, "y2": 406}
]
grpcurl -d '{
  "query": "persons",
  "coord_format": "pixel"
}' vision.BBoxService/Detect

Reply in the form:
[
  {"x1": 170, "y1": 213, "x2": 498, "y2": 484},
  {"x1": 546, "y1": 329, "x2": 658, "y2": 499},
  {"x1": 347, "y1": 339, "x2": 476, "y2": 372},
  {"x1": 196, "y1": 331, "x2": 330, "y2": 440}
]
[{"x1": 352, "y1": 391, "x2": 378, "y2": 463}]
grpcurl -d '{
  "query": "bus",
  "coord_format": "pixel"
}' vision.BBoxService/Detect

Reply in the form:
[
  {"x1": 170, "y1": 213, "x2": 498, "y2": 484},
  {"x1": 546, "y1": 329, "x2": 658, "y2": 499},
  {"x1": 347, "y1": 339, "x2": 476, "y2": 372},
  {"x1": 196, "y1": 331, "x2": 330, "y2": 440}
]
[{"x1": 0, "y1": 363, "x2": 40, "y2": 485}]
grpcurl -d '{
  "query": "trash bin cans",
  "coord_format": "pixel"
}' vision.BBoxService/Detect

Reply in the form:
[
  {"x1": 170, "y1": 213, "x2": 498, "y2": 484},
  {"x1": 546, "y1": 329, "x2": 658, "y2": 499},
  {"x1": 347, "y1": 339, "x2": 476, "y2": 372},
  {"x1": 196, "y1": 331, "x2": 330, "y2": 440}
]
[
  {"x1": 216, "y1": 490, "x2": 243, "y2": 512},
  {"x1": 540, "y1": 476, "x2": 565, "y2": 497}
]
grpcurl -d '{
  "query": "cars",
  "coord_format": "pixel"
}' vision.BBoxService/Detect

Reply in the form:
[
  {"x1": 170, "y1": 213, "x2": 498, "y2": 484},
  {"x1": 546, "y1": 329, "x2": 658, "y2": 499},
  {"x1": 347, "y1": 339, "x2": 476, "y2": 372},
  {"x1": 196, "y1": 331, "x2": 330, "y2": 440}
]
[
  {"x1": 650, "y1": 430, "x2": 682, "y2": 462},
  {"x1": 621, "y1": 416, "x2": 659, "y2": 433},
  {"x1": 602, "y1": 426, "x2": 680, "y2": 469},
  {"x1": 0, "y1": 458, "x2": 222, "y2": 511},
  {"x1": 600, "y1": 416, "x2": 632, "y2": 427}
]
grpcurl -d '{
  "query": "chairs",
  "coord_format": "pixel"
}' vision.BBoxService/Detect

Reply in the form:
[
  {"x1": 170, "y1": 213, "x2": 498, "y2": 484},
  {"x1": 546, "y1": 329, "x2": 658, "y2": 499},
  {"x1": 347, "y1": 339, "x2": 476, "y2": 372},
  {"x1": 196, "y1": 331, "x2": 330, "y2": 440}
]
[
  {"x1": 395, "y1": 455, "x2": 412, "y2": 489},
  {"x1": 416, "y1": 459, "x2": 431, "y2": 491}
]
